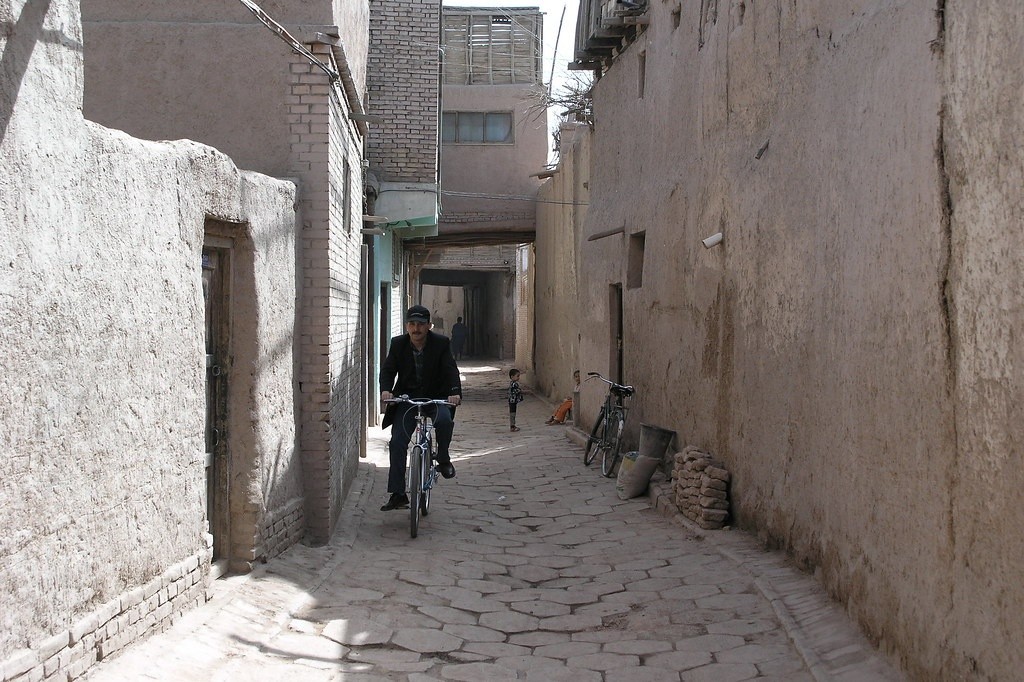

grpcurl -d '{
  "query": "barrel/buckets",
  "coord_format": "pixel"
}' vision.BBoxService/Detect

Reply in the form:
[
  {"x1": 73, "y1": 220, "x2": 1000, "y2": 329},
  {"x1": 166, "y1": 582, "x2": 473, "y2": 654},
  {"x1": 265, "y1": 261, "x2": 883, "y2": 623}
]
[{"x1": 640, "y1": 422, "x2": 674, "y2": 459}]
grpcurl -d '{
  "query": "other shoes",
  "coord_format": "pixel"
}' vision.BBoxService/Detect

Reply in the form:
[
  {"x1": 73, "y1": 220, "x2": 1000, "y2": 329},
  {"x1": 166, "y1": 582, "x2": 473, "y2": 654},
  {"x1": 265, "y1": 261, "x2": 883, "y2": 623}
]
[
  {"x1": 510, "y1": 427, "x2": 520, "y2": 432},
  {"x1": 544, "y1": 416, "x2": 554, "y2": 424},
  {"x1": 549, "y1": 420, "x2": 561, "y2": 425}
]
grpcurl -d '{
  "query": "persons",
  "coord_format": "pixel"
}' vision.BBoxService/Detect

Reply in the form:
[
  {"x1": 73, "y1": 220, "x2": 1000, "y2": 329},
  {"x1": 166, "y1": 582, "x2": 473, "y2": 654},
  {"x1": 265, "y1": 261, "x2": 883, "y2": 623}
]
[
  {"x1": 379, "y1": 304, "x2": 462, "y2": 511},
  {"x1": 452, "y1": 317, "x2": 467, "y2": 360},
  {"x1": 509, "y1": 369, "x2": 522, "y2": 432},
  {"x1": 545, "y1": 370, "x2": 580, "y2": 426}
]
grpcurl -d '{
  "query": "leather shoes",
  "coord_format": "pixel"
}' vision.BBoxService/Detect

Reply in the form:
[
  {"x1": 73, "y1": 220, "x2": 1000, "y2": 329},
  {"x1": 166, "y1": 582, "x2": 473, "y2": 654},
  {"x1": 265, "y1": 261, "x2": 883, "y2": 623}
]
[
  {"x1": 437, "y1": 453, "x2": 455, "y2": 479},
  {"x1": 381, "y1": 492, "x2": 409, "y2": 511}
]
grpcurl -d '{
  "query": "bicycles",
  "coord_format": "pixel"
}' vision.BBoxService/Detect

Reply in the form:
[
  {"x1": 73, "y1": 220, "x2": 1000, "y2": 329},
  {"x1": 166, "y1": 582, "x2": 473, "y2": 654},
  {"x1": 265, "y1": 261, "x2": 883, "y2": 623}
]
[
  {"x1": 383, "y1": 394, "x2": 461, "y2": 538},
  {"x1": 583, "y1": 372, "x2": 634, "y2": 476}
]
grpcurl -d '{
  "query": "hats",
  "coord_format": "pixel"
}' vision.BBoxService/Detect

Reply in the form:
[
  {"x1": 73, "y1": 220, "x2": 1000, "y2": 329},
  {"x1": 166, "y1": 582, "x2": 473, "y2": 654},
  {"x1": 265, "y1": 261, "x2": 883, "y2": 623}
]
[{"x1": 405, "y1": 304, "x2": 430, "y2": 323}]
